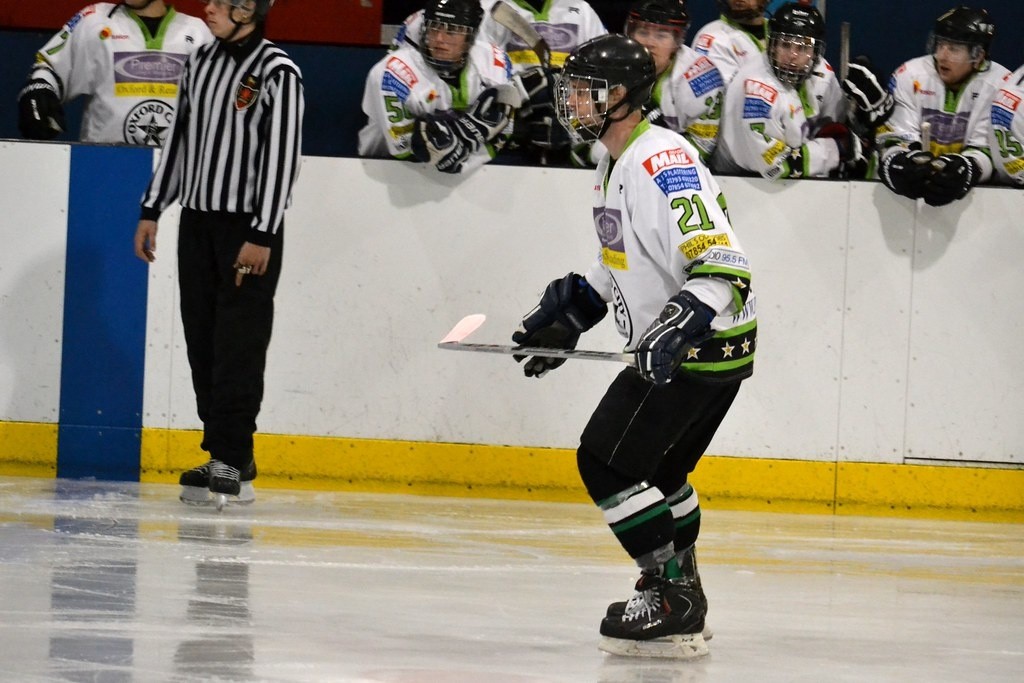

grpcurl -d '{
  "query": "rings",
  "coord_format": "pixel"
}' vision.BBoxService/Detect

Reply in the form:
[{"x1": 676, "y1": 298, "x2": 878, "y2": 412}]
[{"x1": 237, "y1": 262, "x2": 254, "y2": 274}]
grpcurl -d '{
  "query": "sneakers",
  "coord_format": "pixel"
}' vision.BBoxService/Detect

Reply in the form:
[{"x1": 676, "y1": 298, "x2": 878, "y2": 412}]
[
  {"x1": 598, "y1": 569, "x2": 709, "y2": 659},
  {"x1": 210, "y1": 457, "x2": 240, "y2": 512},
  {"x1": 606, "y1": 544, "x2": 713, "y2": 640},
  {"x1": 179, "y1": 453, "x2": 257, "y2": 508}
]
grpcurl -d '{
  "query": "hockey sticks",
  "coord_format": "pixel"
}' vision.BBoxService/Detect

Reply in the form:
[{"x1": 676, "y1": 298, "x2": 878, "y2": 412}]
[
  {"x1": 839, "y1": 20, "x2": 849, "y2": 84},
  {"x1": 921, "y1": 122, "x2": 931, "y2": 152},
  {"x1": 490, "y1": 0, "x2": 552, "y2": 84},
  {"x1": 437, "y1": 314, "x2": 636, "y2": 364}
]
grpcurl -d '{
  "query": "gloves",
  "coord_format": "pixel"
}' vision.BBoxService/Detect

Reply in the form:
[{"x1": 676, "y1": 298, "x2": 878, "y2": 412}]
[
  {"x1": 814, "y1": 123, "x2": 869, "y2": 181},
  {"x1": 18, "y1": 63, "x2": 64, "y2": 136},
  {"x1": 513, "y1": 67, "x2": 560, "y2": 99},
  {"x1": 513, "y1": 272, "x2": 608, "y2": 378},
  {"x1": 410, "y1": 114, "x2": 496, "y2": 174},
  {"x1": 878, "y1": 141, "x2": 935, "y2": 200},
  {"x1": 843, "y1": 56, "x2": 896, "y2": 127},
  {"x1": 518, "y1": 102, "x2": 555, "y2": 147},
  {"x1": 635, "y1": 290, "x2": 717, "y2": 386},
  {"x1": 921, "y1": 153, "x2": 982, "y2": 207},
  {"x1": 452, "y1": 89, "x2": 513, "y2": 153}
]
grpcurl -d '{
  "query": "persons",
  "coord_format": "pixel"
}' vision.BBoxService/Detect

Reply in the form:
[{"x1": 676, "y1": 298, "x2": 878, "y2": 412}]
[
  {"x1": 987, "y1": 63, "x2": 1024, "y2": 184},
  {"x1": 691, "y1": 0, "x2": 775, "y2": 92},
  {"x1": 717, "y1": 2, "x2": 895, "y2": 180},
  {"x1": 475, "y1": 0, "x2": 610, "y2": 170},
  {"x1": 874, "y1": 4, "x2": 1013, "y2": 204},
  {"x1": 624, "y1": 0, "x2": 723, "y2": 159},
  {"x1": 134, "y1": 0, "x2": 305, "y2": 513},
  {"x1": 511, "y1": 33, "x2": 757, "y2": 663},
  {"x1": 358, "y1": 0, "x2": 529, "y2": 177},
  {"x1": 16, "y1": 0, "x2": 217, "y2": 147}
]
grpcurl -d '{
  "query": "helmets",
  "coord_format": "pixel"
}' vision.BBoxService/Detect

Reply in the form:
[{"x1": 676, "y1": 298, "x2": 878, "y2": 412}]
[
  {"x1": 767, "y1": 3, "x2": 827, "y2": 84},
  {"x1": 931, "y1": 4, "x2": 995, "y2": 54},
  {"x1": 554, "y1": 33, "x2": 656, "y2": 143},
  {"x1": 624, "y1": 0, "x2": 692, "y2": 30},
  {"x1": 234, "y1": 0, "x2": 268, "y2": 18},
  {"x1": 420, "y1": 0, "x2": 485, "y2": 74}
]
